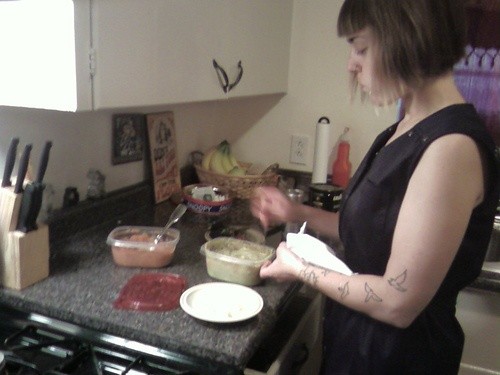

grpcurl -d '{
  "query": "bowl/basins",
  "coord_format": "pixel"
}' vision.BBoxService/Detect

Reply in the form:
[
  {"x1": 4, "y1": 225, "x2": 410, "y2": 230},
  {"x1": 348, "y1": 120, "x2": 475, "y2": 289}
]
[
  {"x1": 183, "y1": 182, "x2": 236, "y2": 216},
  {"x1": 199, "y1": 237, "x2": 276, "y2": 286},
  {"x1": 105, "y1": 224, "x2": 180, "y2": 268}
]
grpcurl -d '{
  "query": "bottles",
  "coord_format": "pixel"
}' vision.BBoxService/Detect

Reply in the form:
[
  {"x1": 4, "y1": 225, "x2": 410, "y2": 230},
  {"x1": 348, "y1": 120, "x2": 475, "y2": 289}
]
[
  {"x1": 311, "y1": 117, "x2": 330, "y2": 184},
  {"x1": 85, "y1": 169, "x2": 106, "y2": 203},
  {"x1": 333, "y1": 142, "x2": 351, "y2": 188},
  {"x1": 62, "y1": 186, "x2": 81, "y2": 209}
]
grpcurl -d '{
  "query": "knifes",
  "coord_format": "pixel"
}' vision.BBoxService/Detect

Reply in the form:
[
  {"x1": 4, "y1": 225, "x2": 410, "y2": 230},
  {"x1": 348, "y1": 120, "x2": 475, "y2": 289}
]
[{"x1": 1, "y1": 136, "x2": 53, "y2": 233}]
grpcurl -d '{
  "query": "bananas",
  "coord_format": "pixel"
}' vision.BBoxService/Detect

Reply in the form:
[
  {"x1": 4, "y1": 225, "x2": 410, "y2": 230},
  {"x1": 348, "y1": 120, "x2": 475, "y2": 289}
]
[{"x1": 200, "y1": 140, "x2": 245, "y2": 176}]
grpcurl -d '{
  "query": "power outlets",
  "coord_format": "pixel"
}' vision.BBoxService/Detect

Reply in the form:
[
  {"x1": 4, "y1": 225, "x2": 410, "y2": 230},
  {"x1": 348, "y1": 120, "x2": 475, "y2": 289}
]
[{"x1": 289, "y1": 134, "x2": 310, "y2": 165}]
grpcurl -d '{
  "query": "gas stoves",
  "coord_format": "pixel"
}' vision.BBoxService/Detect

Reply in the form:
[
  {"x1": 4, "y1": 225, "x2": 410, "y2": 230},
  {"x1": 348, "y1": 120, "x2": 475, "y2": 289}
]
[{"x1": 0, "y1": 300, "x2": 245, "y2": 375}]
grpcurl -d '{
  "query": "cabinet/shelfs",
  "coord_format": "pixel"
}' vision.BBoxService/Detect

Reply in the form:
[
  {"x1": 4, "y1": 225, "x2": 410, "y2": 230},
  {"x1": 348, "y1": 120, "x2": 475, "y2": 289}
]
[
  {"x1": 0, "y1": 0, "x2": 291, "y2": 114},
  {"x1": 243, "y1": 289, "x2": 324, "y2": 375}
]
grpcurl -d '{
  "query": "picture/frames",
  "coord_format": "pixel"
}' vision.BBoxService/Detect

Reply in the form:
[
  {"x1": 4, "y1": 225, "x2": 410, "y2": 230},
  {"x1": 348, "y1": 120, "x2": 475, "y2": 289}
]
[{"x1": 112, "y1": 113, "x2": 148, "y2": 165}]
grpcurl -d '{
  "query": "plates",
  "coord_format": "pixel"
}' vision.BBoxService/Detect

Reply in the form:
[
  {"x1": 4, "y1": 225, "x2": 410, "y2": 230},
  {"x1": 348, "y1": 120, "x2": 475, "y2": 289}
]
[
  {"x1": 204, "y1": 217, "x2": 267, "y2": 245},
  {"x1": 180, "y1": 282, "x2": 263, "y2": 323}
]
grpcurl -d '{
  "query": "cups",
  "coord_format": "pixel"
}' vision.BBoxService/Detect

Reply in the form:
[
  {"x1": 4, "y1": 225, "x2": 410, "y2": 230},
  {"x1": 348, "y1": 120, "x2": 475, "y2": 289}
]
[
  {"x1": 281, "y1": 188, "x2": 303, "y2": 240},
  {"x1": 307, "y1": 182, "x2": 343, "y2": 213}
]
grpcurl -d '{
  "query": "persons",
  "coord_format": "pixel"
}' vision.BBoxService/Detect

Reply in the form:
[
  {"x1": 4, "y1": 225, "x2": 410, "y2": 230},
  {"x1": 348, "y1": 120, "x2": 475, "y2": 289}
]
[{"x1": 249, "y1": 0, "x2": 497, "y2": 375}]
